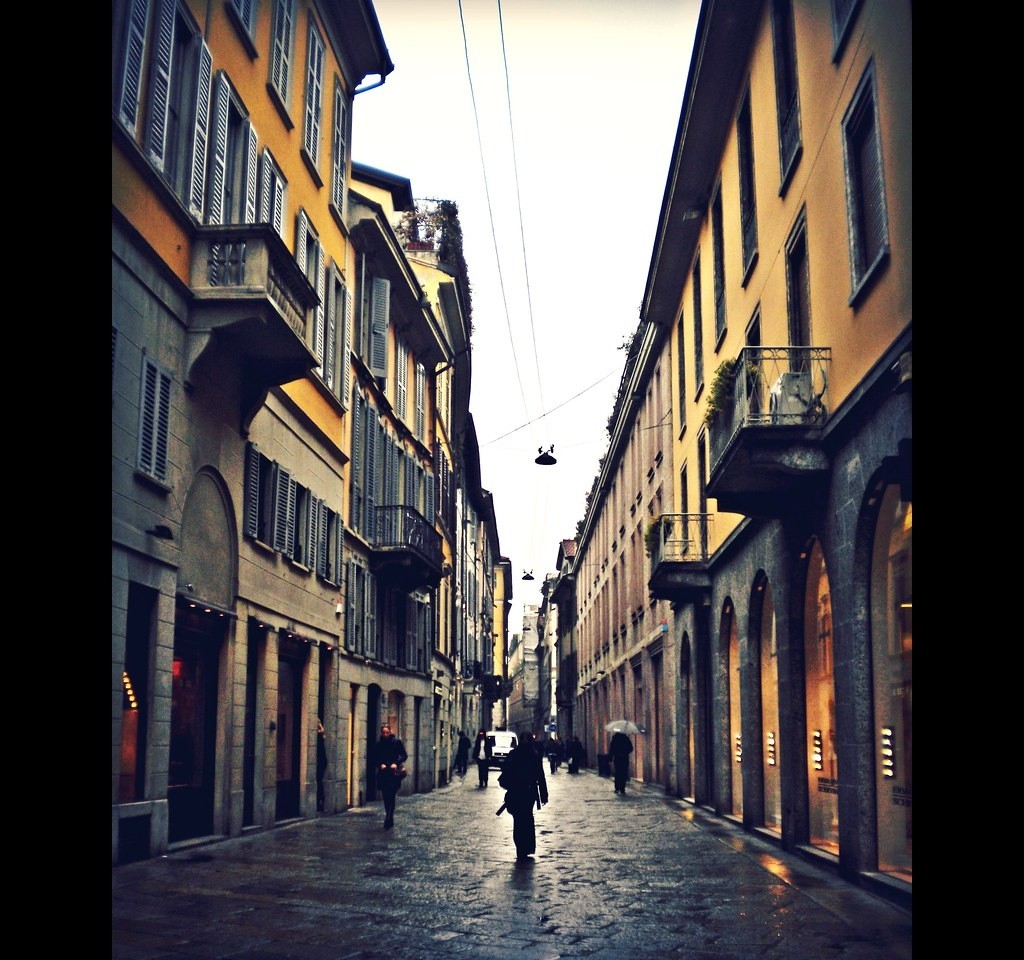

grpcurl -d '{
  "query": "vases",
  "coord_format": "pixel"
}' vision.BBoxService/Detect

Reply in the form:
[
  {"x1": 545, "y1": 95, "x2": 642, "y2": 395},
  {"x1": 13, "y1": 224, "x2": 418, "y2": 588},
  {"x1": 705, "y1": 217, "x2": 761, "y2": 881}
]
[{"x1": 407, "y1": 242, "x2": 434, "y2": 250}]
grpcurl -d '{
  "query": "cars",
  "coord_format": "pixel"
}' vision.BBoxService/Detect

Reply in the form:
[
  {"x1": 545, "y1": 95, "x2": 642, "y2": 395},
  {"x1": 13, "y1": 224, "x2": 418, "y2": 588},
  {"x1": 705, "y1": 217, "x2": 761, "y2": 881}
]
[{"x1": 478, "y1": 729, "x2": 519, "y2": 766}]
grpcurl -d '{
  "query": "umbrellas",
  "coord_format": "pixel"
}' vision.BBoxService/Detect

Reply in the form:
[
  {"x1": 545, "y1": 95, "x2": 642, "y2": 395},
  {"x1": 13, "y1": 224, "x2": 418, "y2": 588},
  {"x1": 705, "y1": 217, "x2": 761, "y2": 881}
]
[{"x1": 605, "y1": 720, "x2": 647, "y2": 735}]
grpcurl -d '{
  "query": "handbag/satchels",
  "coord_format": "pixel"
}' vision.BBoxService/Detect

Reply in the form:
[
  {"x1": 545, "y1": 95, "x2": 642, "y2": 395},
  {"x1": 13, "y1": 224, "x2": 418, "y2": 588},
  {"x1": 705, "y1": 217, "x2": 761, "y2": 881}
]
[{"x1": 393, "y1": 766, "x2": 408, "y2": 779}]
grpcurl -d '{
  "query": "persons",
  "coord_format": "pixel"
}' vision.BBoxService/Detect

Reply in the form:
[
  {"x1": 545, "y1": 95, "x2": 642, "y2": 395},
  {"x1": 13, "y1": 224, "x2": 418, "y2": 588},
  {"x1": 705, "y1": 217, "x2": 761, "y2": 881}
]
[
  {"x1": 609, "y1": 733, "x2": 634, "y2": 795},
  {"x1": 375, "y1": 725, "x2": 408, "y2": 832},
  {"x1": 546, "y1": 736, "x2": 583, "y2": 775},
  {"x1": 501, "y1": 732, "x2": 548, "y2": 863},
  {"x1": 455, "y1": 729, "x2": 492, "y2": 788}
]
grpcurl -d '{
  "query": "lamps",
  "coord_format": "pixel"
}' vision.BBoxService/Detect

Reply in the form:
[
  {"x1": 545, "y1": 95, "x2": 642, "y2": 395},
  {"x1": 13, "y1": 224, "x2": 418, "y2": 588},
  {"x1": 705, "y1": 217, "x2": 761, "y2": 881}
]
[
  {"x1": 522, "y1": 623, "x2": 532, "y2": 631},
  {"x1": 534, "y1": 444, "x2": 558, "y2": 466},
  {"x1": 521, "y1": 569, "x2": 535, "y2": 580}
]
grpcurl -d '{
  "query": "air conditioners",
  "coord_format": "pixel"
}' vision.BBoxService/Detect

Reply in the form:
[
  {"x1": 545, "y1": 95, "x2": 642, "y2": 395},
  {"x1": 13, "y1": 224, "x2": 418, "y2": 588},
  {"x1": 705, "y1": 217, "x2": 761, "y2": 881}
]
[{"x1": 769, "y1": 367, "x2": 828, "y2": 425}]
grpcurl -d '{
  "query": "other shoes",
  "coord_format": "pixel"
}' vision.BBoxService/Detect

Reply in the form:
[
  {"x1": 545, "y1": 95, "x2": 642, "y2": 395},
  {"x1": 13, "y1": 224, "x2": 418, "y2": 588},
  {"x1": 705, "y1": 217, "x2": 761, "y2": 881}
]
[{"x1": 383, "y1": 820, "x2": 395, "y2": 830}]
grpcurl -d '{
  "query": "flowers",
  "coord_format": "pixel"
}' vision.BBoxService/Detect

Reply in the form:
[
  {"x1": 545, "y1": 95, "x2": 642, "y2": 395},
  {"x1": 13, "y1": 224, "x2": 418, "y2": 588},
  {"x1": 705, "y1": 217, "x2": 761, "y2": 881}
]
[{"x1": 393, "y1": 201, "x2": 447, "y2": 248}]
